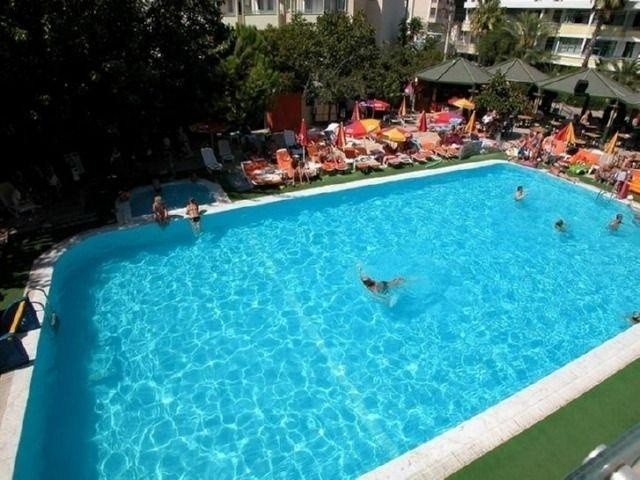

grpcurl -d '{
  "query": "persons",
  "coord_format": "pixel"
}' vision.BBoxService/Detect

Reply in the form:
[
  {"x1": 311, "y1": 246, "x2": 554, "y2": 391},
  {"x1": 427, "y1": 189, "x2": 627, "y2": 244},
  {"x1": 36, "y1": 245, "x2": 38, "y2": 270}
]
[
  {"x1": 632, "y1": 311, "x2": 640, "y2": 323},
  {"x1": 451, "y1": 124, "x2": 465, "y2": 136},
  {"x1": 516, "y1": 186, "x2": 525, "y2": 200},
  {"x1": 517, "y1": 131, "x2": 543, "y2": 159},
  {"x1": 152, "y1": 196, "x2": 168, "y2": 225},
  {"x1": 555, "y1": 219, "x2": 564, "y2": 230},
  {"x1": 364, "y1": 277, "x2": 404, "y2": 293},
  {"x1": 291, "y1": 160, "x2": 311, "y2": 187},
  {"x1": 186, "y1": 198, "x2": 200, "y2": 227},
  {"x1": 245, "y1": 154, "x2": 283, "y2": 185},
  {"x1": 610, "y1": 214, "x2": 623, "y2": 230}
]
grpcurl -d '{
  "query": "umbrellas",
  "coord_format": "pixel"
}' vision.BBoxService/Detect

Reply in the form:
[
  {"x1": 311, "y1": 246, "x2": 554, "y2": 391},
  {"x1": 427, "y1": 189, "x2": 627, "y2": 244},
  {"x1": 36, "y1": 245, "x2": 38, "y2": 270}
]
[
  {"x1": 465, "y1": 110, "x2": 477, "y2": 134},
  {"x1": 295, "y1": 118, "x2": 308, "y2": 161},
  {"x1": 351, "y1": 100, "x2": 360, "y2": 120},
  {"x1": 398, "y1": 96, "x2": 406, "y2": 117},
  {"x1": 377, "y1": 126, "x2": 413, "y2": 143},
  {"x1": 336, "y1": 122, "x2": 346, "y2": 147},
  {"x1": 452, "y1": 98, "x2": 476, "y2": 110},
  {"x1": 418, "y1": 110, "x2": 428, "y2": 132},
  {"x1": 555, "y1": 121, "x2": 576, "y2": 145},
  {"x1": 360, "y1": 99, "x2": 391, "y2": 117},
  {"x1": 344, "y1": 119, "x2": 381, "y2": 138},
  {"x1": 606, "y1": 130, "x2": 619, "y2": 154}
]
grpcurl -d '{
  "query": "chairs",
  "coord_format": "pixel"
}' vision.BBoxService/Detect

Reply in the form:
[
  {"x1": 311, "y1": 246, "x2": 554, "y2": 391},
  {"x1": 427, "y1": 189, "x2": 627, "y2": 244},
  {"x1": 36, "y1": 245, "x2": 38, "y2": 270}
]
[{"x1": 200, "y1": 138, "x2": 426, "y2": 191}]
